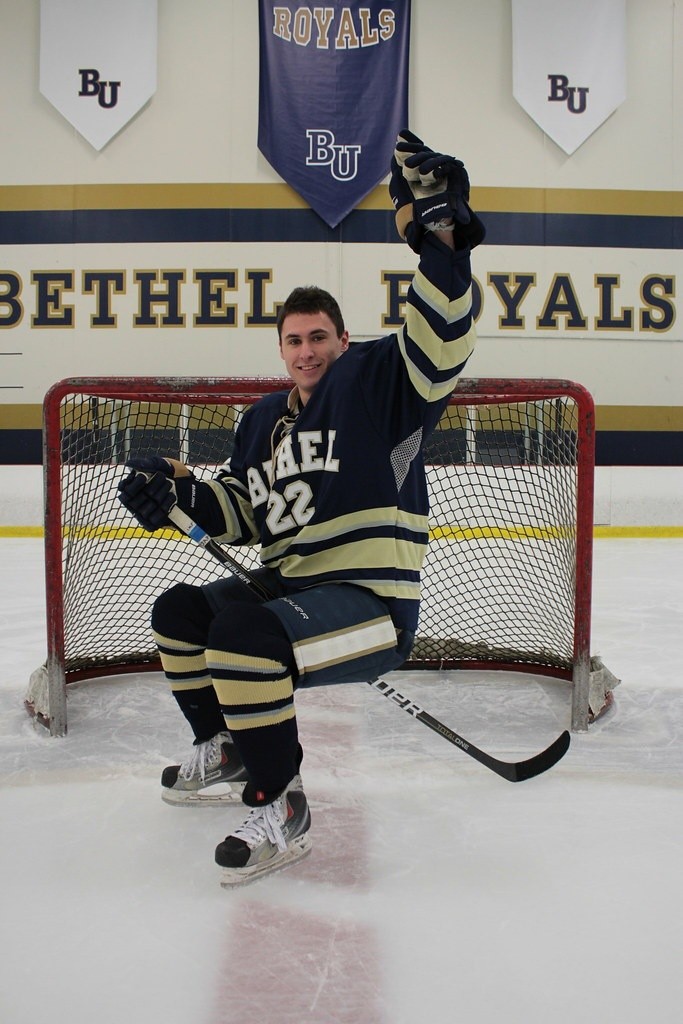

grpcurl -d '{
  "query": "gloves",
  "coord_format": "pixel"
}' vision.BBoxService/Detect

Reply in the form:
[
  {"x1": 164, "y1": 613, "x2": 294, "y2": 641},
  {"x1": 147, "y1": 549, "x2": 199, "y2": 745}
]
[
  {"x1": 118, "y1": 456, "x2": 214, "y2": 542},
  {"x1": 389, "y1": 129, "x2": 487, "y2": 254}
]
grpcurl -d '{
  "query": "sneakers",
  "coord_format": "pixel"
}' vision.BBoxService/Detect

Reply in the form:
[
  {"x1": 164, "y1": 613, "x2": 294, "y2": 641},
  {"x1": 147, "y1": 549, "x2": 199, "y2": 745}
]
[
  {"x1": 162, "y1": 689, "x2": 250, "y2": 808},
  {"x1": 215, "y1": 740, "x2": 313, "y2": 889}
]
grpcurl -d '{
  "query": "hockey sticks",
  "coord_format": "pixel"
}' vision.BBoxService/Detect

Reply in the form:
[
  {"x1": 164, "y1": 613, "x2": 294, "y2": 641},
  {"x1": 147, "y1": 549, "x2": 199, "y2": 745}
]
[{"x1": 137, "y1": 474, "x2": 574, "y2": 784}]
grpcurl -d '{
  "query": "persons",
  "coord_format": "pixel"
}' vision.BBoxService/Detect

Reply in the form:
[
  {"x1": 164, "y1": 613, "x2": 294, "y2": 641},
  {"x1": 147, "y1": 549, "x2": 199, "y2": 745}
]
[{"x1": 117, "y1": 130, "x2": 486, "y2": 870}]
